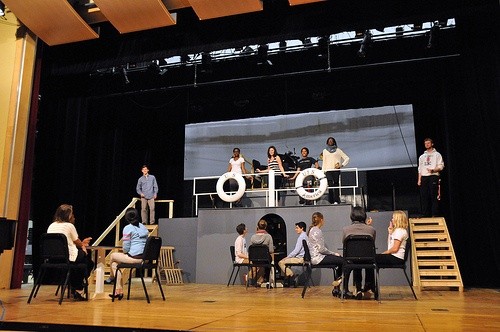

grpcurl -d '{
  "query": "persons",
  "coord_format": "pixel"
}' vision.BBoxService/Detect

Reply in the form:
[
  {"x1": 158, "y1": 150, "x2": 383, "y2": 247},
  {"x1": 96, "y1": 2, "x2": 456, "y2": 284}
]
[
  {"x1": 47, "y1": 205, "x2": 94, "y2": 300},
  {"x1": 342, "y1": 206, "x2": 377, "y2": 298},
  {"x1": 103, "y1": 208, "x2": 148, "y2": 300},
  {"x1": 278, "y1": 222, "x2": 308, "y2": 288},
  {"x1": 289, "y1": 147, "x2": 319, "y2": 206},
  {"x1": 322, "y1": 137, "x2": 350, "y2": 204},
  {"x1": 418, "y1": 138, "x2": 444, "y2": 218},
  {"x1": 136, "y1": 165, "x2": 158, "y2": 225},
  {"x1": 307, "y1": 211, "x2": 353, "y2": 295},
  {"x1": 363, "y1": 210, "x2": 409, "y2": 299},
  {"x1": 234, "y1": 223, "x2": 257, "y2": 286},
  {"x1": 228, "y1": 148, "x2": 252, "y2": 207},
  {"x1": 250, "y1": 220, "x2": 274, "y2": 288},
  {"x1": 255, "y1": 146, "x2": 290, "y2": 205}
]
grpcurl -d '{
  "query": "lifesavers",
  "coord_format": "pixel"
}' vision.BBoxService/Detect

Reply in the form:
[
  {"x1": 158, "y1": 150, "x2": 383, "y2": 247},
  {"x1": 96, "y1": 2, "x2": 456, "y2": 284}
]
[
  {"x1": 216, "y1": 171, "x2": 246, "y2": 203},
  {"x1": 295, "y1": 167, "x2": 328, "y2": 201}
]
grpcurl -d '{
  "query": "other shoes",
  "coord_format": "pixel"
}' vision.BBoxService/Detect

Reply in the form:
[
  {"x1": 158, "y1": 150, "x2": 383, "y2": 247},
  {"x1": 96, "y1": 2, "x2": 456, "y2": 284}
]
[
  {"x1": 249, "y1": 279, "x2": 259, "y2": 287},
  {"x1": 245, "y1": 274, "x2": 248, "y2": 284},
  {"x1": 332, "y1": 290, "x2": 342, "y2": 298},
  {"x1": 283, "y1": 274, "x2": 294, "y2": 286},
  {"x1": 266, "y1": 284, "x2": 272, "y2": 289},
  {"x1": 363, "y1": 286, "x2": 374, "y2": 292},
  {"x1": 75, "y1": 292, "x2": 86, "y2": 300},
  {"x1": 344, "y1": 292, "x2": 356, "y2": 299},
  {"x1": 356, "y1": 292, "x2": 362, "y2": 300}
]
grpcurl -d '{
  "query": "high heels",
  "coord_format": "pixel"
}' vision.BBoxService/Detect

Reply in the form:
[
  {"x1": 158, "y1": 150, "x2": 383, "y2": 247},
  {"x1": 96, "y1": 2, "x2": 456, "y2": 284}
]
[
  {"x1": 109, "y1": 293, "x2": 123, "y2": 300},
  {"x1": 104, "y1": 278, "x2": 115, "y2": 284}
]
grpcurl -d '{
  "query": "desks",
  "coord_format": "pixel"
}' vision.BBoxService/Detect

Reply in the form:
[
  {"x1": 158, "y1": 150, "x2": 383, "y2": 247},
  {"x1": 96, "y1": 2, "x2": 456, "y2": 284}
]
[
  {"x1": 339, "y1": 246, "x2": 379, "y2": 298},
  {"x1": 248, "y1": 252, "x2": 284, "y2": 288},
  {"x1": 83, "y1": 246, "x2": 123, "y2": 299}
]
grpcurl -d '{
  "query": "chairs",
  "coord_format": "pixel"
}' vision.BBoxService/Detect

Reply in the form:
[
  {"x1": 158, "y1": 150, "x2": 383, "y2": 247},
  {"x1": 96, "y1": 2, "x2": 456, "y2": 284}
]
[
  {"x1": 26, "y1": 233, "x2": 89, "y2": 305},
  {"x1": 114, "y1": 236, "x2": 165, "y2": 303},
  {"x1": 248, "y1": 242, "x2": 273, "y2": 287},
  {"x1": 376, "y1": 241, "x2": 416, "y2": 300},
  {"x1": 285, "y1": 261, "x2": 314, "y2": 289},
  {"x1": 343, "y1": 234, "x2": 375, "y2": 302},
  {"x1": 302, "y1": 240, "x2": 341, "y2": 298},
  {"x1": 153, "y1": 245, "x2": 183, "y2": 285},
  {"x1": 227, "y1": 245, "x2": 253, "y2": 287}
]
